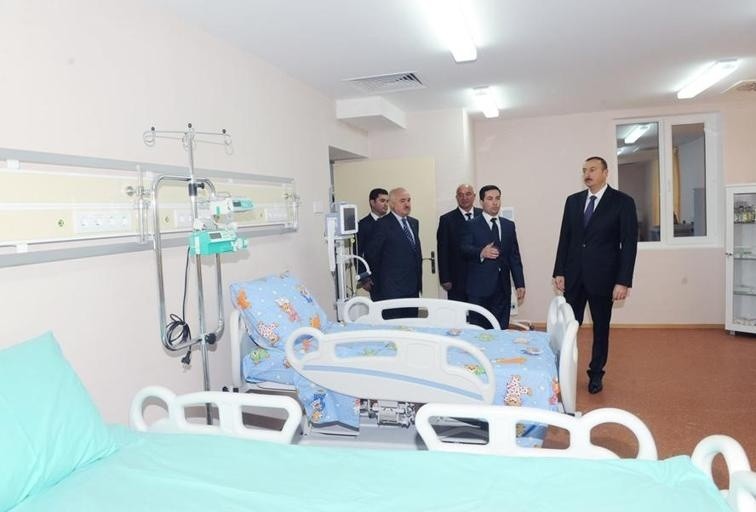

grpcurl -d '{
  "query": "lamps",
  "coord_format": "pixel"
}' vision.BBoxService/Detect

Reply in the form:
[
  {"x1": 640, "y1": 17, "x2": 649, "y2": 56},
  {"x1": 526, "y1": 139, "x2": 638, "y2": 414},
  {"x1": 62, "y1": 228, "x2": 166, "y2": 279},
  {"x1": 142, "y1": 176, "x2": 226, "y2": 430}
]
[
  {"x1": 623, "y1": 123, "x2": 650, "y2": 144},
  {"x1": 445, "y1": 27, "x2": 741, "y2": 118}
]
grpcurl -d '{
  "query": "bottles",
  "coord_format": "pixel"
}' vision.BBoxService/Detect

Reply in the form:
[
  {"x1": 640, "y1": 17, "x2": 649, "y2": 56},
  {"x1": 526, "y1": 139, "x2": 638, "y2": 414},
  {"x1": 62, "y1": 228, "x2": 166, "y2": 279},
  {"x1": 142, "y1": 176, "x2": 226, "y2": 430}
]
[
  {"x1": 510, "y1": 287, "x2": 519, "y2": 315},
  {"x1": 734, "y1": 199, "x2": 756, "y2": 222}
]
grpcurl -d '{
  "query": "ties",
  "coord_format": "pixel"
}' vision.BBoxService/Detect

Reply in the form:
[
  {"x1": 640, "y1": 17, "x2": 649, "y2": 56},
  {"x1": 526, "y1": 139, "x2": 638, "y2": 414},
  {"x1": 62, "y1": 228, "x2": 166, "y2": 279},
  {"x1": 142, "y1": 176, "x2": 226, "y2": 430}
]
[
  {"x1": 464, "y1": 213, "x2": 471, "y2": 219},
  {"x1": 401, "y1": 219, "x2": 412, "y2": 242},
  {"x1": 490, "y1": 218, "x2": 499, "y2": 245},
  {"x1": 583, "y1": 196, "x2": 596, "y2": 230}
]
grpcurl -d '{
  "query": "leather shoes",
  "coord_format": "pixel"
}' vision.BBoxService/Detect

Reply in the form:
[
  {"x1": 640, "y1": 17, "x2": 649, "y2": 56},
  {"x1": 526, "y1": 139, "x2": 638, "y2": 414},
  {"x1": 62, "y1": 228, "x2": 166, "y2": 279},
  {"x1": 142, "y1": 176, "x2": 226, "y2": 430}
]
[{"x1": 588, "y1": 377, "x2": 602, "y2": 393}]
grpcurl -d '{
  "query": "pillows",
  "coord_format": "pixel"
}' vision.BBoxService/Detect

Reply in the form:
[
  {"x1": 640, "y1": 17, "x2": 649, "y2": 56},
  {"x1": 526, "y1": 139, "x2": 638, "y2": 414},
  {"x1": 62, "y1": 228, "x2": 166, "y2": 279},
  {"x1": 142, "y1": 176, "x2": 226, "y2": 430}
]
[
  {"x1": 2, "y1": 329, "x2": 119, "y2": 512},
  {"x1": 229, "y1": 275, "x2": 328, "y2": 350}
]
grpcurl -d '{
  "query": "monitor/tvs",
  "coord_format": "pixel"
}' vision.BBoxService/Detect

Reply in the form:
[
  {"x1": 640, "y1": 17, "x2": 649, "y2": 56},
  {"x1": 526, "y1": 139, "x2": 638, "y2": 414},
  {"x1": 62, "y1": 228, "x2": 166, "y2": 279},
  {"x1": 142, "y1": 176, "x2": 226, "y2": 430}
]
[{"x1": 324, "y1": 203, "x2": 358, "y2": 236}]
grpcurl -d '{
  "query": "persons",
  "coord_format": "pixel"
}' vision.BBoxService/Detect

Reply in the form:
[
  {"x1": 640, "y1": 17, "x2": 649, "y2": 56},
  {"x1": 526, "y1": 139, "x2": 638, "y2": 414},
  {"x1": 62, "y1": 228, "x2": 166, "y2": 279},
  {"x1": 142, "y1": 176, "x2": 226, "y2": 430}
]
[
  {"x1": 436, "y1": 182, "x2": 486, "y2": 304},
  {"x1": 452, "y1": 185, "x2": 526, "y2": 331},
  {"x1": 353, "y1": 188, "x2": 388, "y2": 291},
  {"x1": 361, "y1": 187, "x2": 424, "y2": 320},
  {"x1": 552, "y1": 157, "x2": 639, "y2": 394}
]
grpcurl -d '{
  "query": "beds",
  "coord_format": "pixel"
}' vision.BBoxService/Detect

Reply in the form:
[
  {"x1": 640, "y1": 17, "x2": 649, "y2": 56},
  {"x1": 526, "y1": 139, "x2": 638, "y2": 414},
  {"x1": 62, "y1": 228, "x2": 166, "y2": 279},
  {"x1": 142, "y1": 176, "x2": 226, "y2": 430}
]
[
  {"x1": 2, "y1": 386, "x2": 756, "y2": 512},
  {"x1": 230, "y1": 297, "x2": 580, "y2": 446}
]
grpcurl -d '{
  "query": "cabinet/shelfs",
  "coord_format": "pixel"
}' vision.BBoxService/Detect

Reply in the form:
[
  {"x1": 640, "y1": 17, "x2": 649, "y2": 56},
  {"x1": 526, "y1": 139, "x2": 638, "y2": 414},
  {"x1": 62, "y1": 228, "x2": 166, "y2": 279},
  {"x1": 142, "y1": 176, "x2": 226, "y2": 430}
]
[{"x1": 724, "y1": 182, "x2": 756, "y2": 336}]
